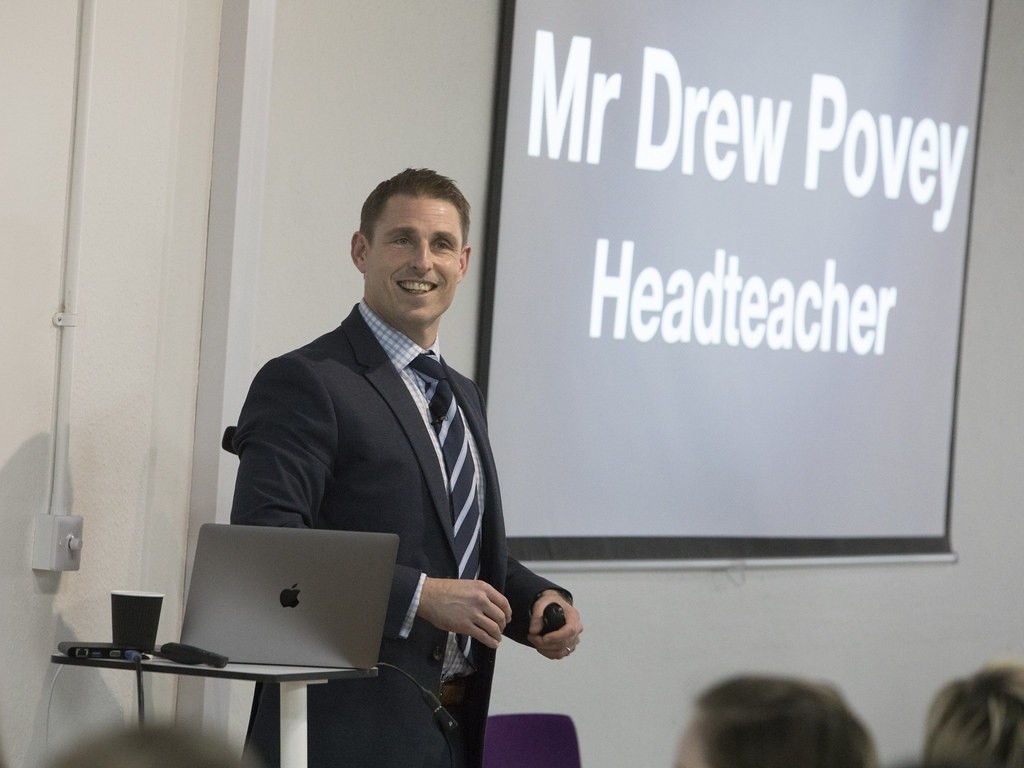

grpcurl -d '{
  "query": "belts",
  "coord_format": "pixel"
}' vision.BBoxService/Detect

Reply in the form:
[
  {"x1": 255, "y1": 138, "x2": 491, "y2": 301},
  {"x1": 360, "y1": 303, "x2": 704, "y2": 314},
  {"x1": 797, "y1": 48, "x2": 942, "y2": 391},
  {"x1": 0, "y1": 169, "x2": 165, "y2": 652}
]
[{"x1": 442, "y1": 671, "x2": 479, "y2": 704}]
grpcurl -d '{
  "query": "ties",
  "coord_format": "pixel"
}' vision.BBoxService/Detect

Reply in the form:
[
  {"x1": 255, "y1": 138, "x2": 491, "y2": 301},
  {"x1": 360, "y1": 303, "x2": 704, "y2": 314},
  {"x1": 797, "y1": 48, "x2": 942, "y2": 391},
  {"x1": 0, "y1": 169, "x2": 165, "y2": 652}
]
[{"x1": 410, "y1": 353, "x2": 482, "y2": 673}]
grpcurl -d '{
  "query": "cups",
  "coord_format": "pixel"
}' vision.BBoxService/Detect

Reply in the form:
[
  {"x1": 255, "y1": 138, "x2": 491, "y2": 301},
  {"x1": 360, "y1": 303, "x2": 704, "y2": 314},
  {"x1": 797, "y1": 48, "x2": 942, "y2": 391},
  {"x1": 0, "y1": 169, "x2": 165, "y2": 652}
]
[{"x1": 111, "y1": 590, "x2": 165, "y2": 650}]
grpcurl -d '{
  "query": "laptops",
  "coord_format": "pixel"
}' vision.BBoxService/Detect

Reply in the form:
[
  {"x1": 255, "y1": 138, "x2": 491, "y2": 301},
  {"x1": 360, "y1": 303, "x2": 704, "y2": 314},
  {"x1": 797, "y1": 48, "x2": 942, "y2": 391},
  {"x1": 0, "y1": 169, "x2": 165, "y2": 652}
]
[{"x1": 149, "y1": 523, "x2": 401, "y2": 669}]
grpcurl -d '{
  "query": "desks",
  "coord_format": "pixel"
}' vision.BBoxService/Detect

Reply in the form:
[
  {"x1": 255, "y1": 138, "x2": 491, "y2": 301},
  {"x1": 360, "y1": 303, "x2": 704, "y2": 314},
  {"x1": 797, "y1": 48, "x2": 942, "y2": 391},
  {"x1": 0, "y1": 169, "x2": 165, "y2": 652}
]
[{"x1": 51, "y1": 652, "x2": 378, "y2": 768}]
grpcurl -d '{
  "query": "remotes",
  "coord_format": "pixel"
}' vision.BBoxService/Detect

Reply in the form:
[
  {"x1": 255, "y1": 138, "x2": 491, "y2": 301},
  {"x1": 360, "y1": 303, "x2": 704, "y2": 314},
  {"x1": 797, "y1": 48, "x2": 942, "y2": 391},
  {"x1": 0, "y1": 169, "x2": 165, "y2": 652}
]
[
  {"x1": 543, "y1": 602, "x2": 566, "y2": 633},
  {"x1": 161, "y1": 643, "x2": 228, "y2": 669}
]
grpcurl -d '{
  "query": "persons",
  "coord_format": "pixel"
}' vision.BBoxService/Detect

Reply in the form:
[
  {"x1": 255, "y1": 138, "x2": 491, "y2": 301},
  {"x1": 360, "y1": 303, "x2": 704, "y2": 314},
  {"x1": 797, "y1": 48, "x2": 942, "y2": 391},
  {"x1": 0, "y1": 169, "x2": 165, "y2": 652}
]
[
  {"x1": 56, "y1": 727, "x2": 242, "y2": 768},
  {"x1": 229, "y1": 168, "x2": 583, "y2": 768},
  {"x1": 924, "y1": 661, "x2": 1024, "y2": 768},
  {"x1": 677, "y1": 674, "x2": 872, "y2": 768}
]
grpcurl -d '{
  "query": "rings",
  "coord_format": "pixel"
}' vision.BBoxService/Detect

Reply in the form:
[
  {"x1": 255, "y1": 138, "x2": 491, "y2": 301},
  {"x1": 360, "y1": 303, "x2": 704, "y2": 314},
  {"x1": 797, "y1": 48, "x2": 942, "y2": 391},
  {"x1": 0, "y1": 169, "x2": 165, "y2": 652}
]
[{"x1": 566, "y1": 646, "x2": 571, "y2": 652}]
